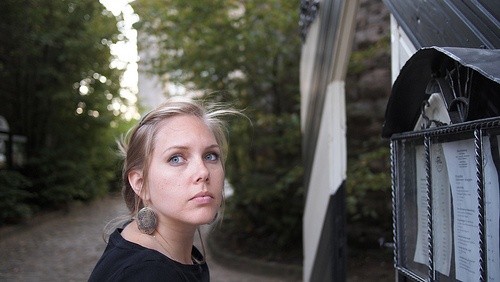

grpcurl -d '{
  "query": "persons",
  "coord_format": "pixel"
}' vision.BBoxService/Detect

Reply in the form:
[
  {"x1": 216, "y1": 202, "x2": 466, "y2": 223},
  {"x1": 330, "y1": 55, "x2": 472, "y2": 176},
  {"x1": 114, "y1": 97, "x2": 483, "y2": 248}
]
[{"x1": 87, "y1": 95, "x2": 254, "y2": 282}]
